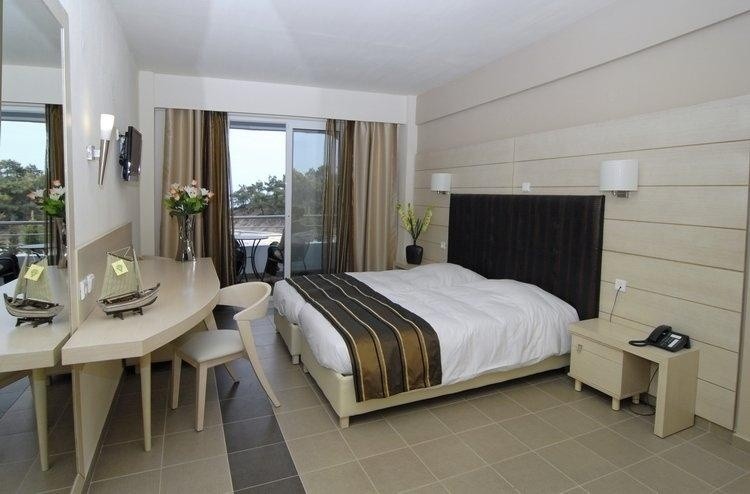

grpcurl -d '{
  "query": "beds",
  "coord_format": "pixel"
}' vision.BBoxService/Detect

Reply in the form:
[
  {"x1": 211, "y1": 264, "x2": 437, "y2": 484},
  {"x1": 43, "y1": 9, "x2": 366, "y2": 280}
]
[{"x1": 273, "y1": 193, "x2": 605, "y2": 428}]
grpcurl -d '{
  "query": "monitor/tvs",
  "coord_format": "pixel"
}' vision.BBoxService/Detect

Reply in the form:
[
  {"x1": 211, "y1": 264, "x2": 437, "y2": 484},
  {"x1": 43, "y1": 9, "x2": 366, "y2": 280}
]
[{"x1": 119, "y1": 125, "x2": 142, "y2": 181}]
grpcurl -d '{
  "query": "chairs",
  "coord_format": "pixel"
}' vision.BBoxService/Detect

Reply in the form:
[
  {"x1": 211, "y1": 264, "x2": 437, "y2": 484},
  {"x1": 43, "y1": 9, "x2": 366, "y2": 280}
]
[
  {"x1": 0, "y1": 254, "x2": 20, "y2": 284},
  {"x1": 262, "y1": 222, "x2": 313, "y2": 283},
  {"x1": 169, "y1": 281, "x2": 282, "y2": 431}
]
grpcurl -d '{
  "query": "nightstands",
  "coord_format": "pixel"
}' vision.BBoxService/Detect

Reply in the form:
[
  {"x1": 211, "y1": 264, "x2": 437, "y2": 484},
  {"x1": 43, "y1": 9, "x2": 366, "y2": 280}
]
[
  {"x1": 565, "y1": 318, "x2": 700, "y2": 438},
  {"x1": 393, "y1": 259, "x2": 433, "y2": 271}
]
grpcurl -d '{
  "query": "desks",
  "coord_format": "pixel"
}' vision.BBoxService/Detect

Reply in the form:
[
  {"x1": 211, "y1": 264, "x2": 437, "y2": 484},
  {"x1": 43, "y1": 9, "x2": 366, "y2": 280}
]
[
  {"x1": 61, "y1": 256, "x2": 221, "y2": 452},
  {"x1": 233, "y1": 234, "x2": 268, "y2": 282},
  {"x1": 0, "y1": 265, "x2": 69, "y2": 473}
]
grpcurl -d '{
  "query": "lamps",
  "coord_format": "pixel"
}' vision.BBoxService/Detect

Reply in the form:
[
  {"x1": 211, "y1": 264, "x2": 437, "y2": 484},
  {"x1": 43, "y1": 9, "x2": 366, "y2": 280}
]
[
  {"x1": 598, "y1": 157, "x2": 638, "y2": 198},
  {"x1": 86, "y1": 113, "x2": 115, "y2": 185},
  {"x1": 430, "y1": 173, "x2": 451, "y2": 196}
]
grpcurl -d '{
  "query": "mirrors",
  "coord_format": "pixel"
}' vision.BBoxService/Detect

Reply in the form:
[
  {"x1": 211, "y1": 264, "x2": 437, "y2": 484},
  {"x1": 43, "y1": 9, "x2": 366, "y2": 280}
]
[{"x1": 0, "y1": 1, "x2": 86, "y2": 493}]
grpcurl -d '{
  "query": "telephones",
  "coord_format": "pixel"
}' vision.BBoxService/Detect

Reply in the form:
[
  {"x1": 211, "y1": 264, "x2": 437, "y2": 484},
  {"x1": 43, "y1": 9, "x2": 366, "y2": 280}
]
[{"x1": 644, "y1": 325, "x2": 691, "y2": 353}]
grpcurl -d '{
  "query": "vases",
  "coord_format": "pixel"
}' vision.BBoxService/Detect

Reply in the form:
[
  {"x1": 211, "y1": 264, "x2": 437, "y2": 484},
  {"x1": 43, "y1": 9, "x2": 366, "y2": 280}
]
[
  {"x1": 405, "y1": 244, "x2": 424, "y2": 265},
  {"x1": 55, "y1": 217, "x2": 68, "y2": 270},
  {"x1": 174, "y1": 213, "x2": 197, "y2": 262}
]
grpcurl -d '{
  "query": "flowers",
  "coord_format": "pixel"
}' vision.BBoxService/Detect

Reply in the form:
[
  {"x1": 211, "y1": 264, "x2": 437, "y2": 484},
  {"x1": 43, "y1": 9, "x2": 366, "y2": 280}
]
[
  {"x1": 25, "y1": 179, "x2": 66, "y2": 218},
  {"x1": 164, "y1": 180, "x2": 214, "y2": 219},
  {"x1": 394, "y1": 200, "x2": 433, "y2": 246}
]
